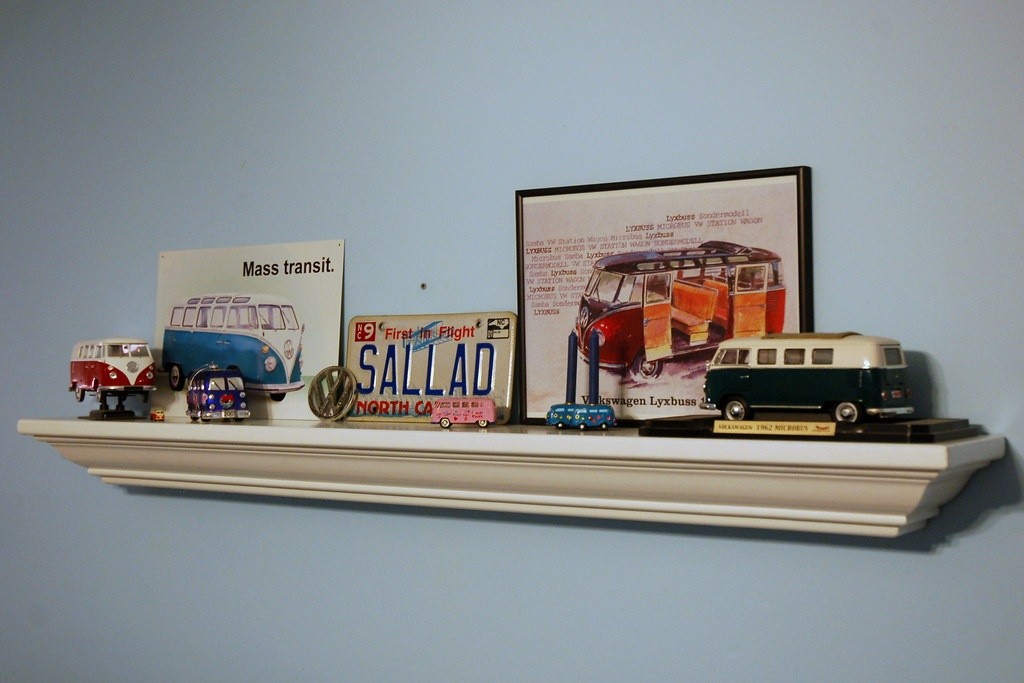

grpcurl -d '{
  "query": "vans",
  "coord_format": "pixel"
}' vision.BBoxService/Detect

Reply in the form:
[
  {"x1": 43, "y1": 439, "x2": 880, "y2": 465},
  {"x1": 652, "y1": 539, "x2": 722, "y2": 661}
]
[
  {"x1": 182, "y1": 361, "x2": 251, "y2": 424},
  {"x1": 571, "y1": 405, "x2": 616, "y2": 432},
  {"x1": 150, "y1": 407, "x2": 165, "y2": 421},
  {"x1": 545, "y1": 403, "x2": 579, "y2": 429}
]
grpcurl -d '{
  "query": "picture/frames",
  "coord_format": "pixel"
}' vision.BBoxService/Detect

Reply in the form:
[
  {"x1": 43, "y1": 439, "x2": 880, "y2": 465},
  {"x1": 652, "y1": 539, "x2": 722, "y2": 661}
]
[{"x1": 514, "y1": 166, "x2": 813, "y2": 426}]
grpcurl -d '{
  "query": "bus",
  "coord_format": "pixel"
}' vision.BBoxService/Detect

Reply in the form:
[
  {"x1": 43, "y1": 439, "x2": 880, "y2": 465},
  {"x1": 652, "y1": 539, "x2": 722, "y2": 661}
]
[
  {"x1": 695, "y1": 331, "x2": 917, "y2": 424},
  {"x1": 66, "y1": 339, "x2": 157, "y2": 404},
  {"x1": 427, "y1": 396, "x2": 497, "y2": 428}
]
